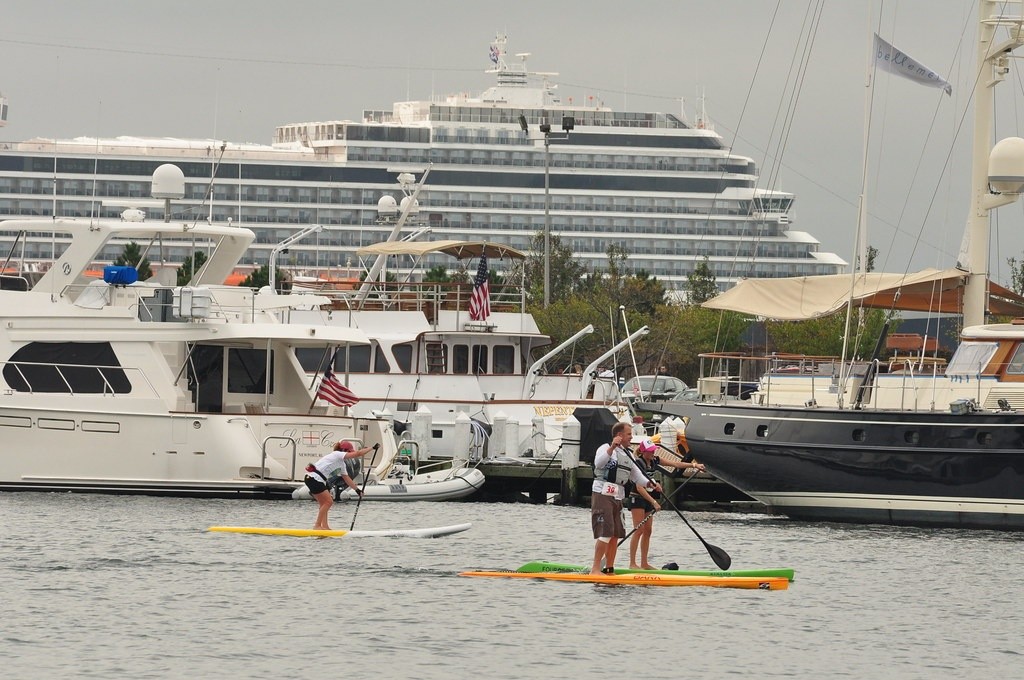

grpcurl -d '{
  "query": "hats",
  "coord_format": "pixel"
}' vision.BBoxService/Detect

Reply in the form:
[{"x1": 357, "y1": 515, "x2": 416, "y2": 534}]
[
  {"x1": 338, "y1": 441, "x2": 355, "y2": 452},
  {"x1": 639, "y1": 439, "x2": 660, "y2": 452}
]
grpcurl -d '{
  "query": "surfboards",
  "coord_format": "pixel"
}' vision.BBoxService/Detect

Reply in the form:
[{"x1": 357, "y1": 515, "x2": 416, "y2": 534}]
[
  {"x1": 456, "y1": 572, "x2": 789, "y2": 591},
  {"x1": 515, "y1": 562, "x2": 794, "y2": 581},
  {"x1": 207, "y1": 522, "x2": 473, "y2": 537}
]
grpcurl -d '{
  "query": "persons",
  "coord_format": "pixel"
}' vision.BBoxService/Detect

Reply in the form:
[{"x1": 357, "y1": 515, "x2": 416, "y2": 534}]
[
  {"x1": 660, "y1": 366, "x2": 669, "y2": 377},
  {"x1": 590, "y1": 422, "x2": 659, "y2": 578},
  {"x1": 626, "y1": 438, "x2": 705, "y2": 573},
  {"x1": 305, "y1": 439, "x2": 381, "y2": 529}
]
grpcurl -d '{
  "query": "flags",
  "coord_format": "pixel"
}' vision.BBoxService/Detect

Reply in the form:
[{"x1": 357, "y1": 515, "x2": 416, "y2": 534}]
[
  {"x1": 870, "y1": 36, "x2": 956, "y2": 97},
  {"x1": 469, "y1": 248, "x2": 490, "y2": 323},
  {"x1": 315, "y1": 368, "x2": 360, "y2": 409}
]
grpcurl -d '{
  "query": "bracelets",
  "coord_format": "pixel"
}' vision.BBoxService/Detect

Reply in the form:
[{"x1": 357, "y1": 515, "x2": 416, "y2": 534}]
[{"x1": 691, "y1": 463, "x2": 696, "y2": 467}]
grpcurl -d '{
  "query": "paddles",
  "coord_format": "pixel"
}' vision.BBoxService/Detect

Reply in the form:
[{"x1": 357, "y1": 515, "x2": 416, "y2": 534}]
[
  {"x1": 591, "y1": 463, "x2": 706, "y2": 571},
  {"x1": 349, "y1": 444, "x2": 380, "y2": 532},
  {"x1": 614, "y1": 438, "x2": 731, "y2": 570}
]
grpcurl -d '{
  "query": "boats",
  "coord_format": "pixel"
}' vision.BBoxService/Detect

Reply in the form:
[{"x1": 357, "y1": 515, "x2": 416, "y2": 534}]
[
  {"x1": 290, "y1": 467, "x2": 485, "y2": 501},
  {"x1": 0, "y1": 164, "x2": 1022, "y2": 531}
]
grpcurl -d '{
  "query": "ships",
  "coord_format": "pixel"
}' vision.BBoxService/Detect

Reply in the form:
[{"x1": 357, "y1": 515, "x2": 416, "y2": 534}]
[{"x1": 1, "y1": 37, "x2": 846, "y2": 308}]
